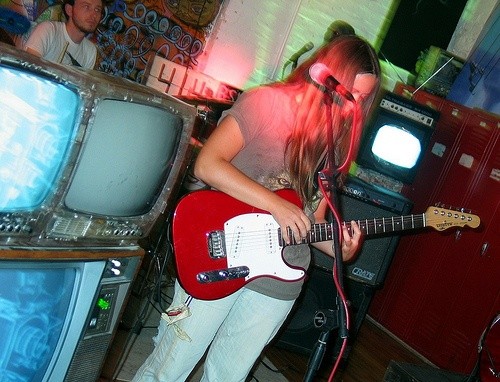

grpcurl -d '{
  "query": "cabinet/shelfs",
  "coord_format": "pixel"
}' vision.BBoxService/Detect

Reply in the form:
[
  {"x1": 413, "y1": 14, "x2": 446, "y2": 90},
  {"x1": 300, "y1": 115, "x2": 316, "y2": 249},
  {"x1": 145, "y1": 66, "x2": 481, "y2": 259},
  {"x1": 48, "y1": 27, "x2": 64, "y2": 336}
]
[{"x1": 356, "y1": 81, "x2": 500, "y2": 382}]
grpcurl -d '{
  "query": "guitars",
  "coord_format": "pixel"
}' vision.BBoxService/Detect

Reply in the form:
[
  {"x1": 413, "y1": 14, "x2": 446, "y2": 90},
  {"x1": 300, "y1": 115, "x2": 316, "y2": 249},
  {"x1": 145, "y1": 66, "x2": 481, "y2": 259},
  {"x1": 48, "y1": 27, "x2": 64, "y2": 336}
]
[{"x1": 168, "y1": 188, "x2": 482, "y2": 301}]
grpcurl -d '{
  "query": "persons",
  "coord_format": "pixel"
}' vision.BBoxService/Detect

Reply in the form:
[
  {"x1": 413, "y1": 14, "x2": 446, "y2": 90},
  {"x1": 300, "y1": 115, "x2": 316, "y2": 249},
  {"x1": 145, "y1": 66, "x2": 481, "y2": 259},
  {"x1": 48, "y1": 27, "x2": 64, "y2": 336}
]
[
  {"x1": 24, "y1": 0, "x2": 103, "y2": 69},
  {"x1": 132, "y1": 35, "x2": 382, "y2": 382}
]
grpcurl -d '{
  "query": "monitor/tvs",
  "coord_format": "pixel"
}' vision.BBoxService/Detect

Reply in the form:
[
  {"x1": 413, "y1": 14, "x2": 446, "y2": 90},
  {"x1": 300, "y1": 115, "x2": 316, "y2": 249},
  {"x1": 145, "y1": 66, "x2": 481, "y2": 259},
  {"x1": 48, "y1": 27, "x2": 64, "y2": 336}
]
[
  {"x1": 43, "y1": 65, "x2": 198, "y2": 243},
  {"x1": 0, "y1": 255, "x2": 141, "y2": 382},
  {"x1": 0, "y1": 42, "x2": 96, "y2": 237},
  {"x1": 355, "y1": 90, "x2": 439, "y2": 184}
]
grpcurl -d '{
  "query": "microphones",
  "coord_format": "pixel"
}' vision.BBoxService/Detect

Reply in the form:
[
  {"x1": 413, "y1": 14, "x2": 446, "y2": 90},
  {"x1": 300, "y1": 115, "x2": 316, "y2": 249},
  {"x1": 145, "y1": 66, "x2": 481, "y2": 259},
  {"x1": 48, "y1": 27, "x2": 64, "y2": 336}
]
[
  {"x1": 284, "y1": 42, "x2": 314, "y2": 67},
  {"x1": 309, "y1": 62, "x2": 355, "y2": 101}
]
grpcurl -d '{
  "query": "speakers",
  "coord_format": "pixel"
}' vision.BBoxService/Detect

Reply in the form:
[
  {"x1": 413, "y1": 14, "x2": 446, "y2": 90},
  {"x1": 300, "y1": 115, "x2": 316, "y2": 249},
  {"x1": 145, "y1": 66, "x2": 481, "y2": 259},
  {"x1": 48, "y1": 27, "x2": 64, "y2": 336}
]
[
  {"x1": 271, "y1": 267, "x2": 374, "y2": 368},
  {"x1": 309, "y1": 176, "x2": 413, "y2": 289}
]
